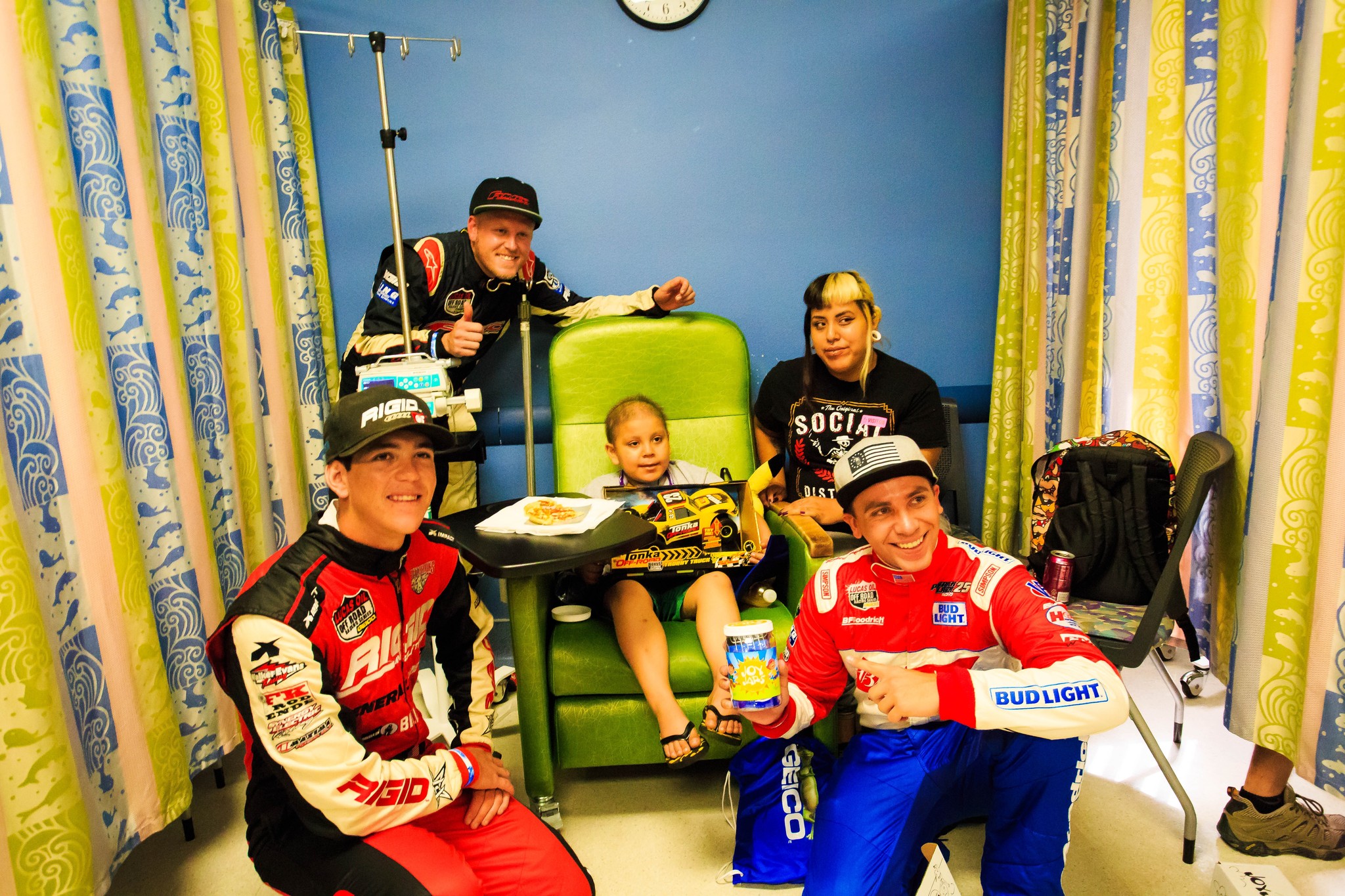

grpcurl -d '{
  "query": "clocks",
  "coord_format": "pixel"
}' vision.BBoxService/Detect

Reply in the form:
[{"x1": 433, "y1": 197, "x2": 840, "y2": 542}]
[{"x1": 616, "y1": 0, "x2": 710, "y2": 31}]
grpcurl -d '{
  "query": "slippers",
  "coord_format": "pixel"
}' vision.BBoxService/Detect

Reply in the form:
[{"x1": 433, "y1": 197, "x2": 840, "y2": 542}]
[
  {"x1": 699, "y1": 705, "x2": 742, "y2": 746},
  {"x1": 659, "y1": 721, "x2": 709, "y2": 763}
]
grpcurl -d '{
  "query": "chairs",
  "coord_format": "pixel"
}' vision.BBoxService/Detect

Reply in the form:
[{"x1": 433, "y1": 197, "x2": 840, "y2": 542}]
[
  {"x1": 1067, "y1": 431, "x2": 1235, "y2": 865},
  {"x1": 507, "y1": 309, "x2": 841, "y2": 804}
]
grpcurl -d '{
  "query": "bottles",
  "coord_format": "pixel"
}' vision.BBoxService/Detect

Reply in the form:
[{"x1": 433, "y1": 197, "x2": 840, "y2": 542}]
[
  {"x1": 738, "y1": 580, "x2": 777, "y2": 608},
  {"x1": 723, "y1": 621, "x2": 782, "y2": 711}
]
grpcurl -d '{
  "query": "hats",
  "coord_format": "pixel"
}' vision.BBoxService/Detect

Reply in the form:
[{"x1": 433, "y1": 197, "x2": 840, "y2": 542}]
[
  {"x1": 322, "y1": 387, "x2": 453, "y2": 465},
  {"x1": 835, "y1": 435, "x2": 938, "y2": 509},
  {"x1": 469, "y1": 176, "x2": 543, "y2": 230}
]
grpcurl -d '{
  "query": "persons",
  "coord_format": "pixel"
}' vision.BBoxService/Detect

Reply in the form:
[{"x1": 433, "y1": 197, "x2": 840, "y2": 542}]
[
  {"x1": 717, "y1": 436, "x2": 1131, "y2": 896},
  {"x1": 204, "y1": 384, "x2": 596, "y2": 896},
  {"x1": 577, "y1": 395, "x2": 772, "y2": 766},
  {"x1": 337, "y1": 176, "x2": 696, "y2": 589},
  {"x1": 1216, "y1": 743, "x2": 1345, "y2": 861},
  {"x1": 753, "y1": 272, "x2": 950, "y2": 556}
]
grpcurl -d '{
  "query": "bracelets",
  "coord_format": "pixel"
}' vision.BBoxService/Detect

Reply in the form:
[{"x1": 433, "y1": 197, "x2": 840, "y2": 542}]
[{"x1": 447, "y1": 746, "x2": 480, "y2": 788}]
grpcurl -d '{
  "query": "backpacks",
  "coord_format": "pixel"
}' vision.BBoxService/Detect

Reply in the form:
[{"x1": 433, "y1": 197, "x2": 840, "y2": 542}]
[{"x1": 723, "y1": 728, "x2": 843, "y2": 886}]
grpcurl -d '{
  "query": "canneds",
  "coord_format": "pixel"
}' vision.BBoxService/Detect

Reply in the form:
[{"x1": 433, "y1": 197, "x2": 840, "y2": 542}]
[
  {"x1": 722, "y1": 619, "x2": 782, "y2": 712},
  {"x1": 1042, "y1": 551, "x2": 1075, "y2": 605}
]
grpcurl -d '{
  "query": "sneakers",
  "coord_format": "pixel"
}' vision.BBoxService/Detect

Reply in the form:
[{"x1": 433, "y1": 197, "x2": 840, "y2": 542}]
[{"x1": 1216, "y1": 783, "x2": 1344, "y2": 861}]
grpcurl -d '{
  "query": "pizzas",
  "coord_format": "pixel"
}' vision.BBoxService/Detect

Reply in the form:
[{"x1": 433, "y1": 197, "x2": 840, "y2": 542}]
[{"x1": 523, "y1": 498, "x2": 578, "y2": 525}]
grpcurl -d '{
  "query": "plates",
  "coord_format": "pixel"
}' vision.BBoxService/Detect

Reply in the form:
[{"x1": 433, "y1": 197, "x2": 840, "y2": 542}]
[{"x1": 551, "y1": 604, "x2": 591, "y2": 622}]
